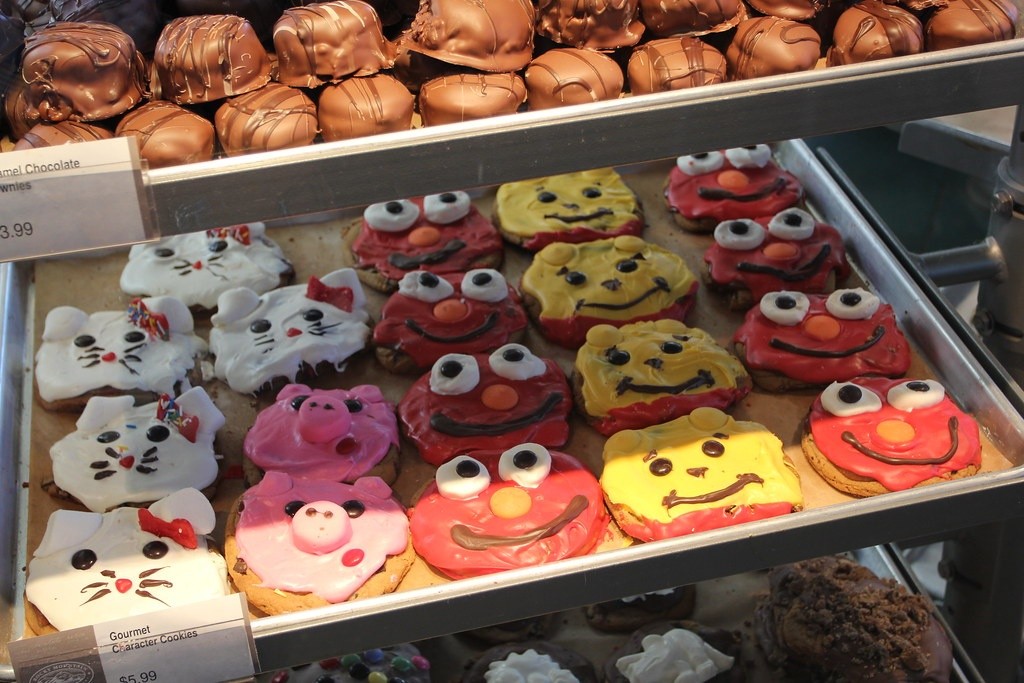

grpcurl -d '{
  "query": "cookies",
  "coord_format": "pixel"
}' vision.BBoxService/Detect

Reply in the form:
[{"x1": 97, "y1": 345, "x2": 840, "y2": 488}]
[{"x1": 29, "y1": 141, "x2": 982, "y2": 683}]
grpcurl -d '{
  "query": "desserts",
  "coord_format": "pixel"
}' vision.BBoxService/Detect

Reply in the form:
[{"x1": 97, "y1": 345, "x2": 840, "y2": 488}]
[{"x1": 0, "y1": 0, "x2": 1024, "y2": 171}]
